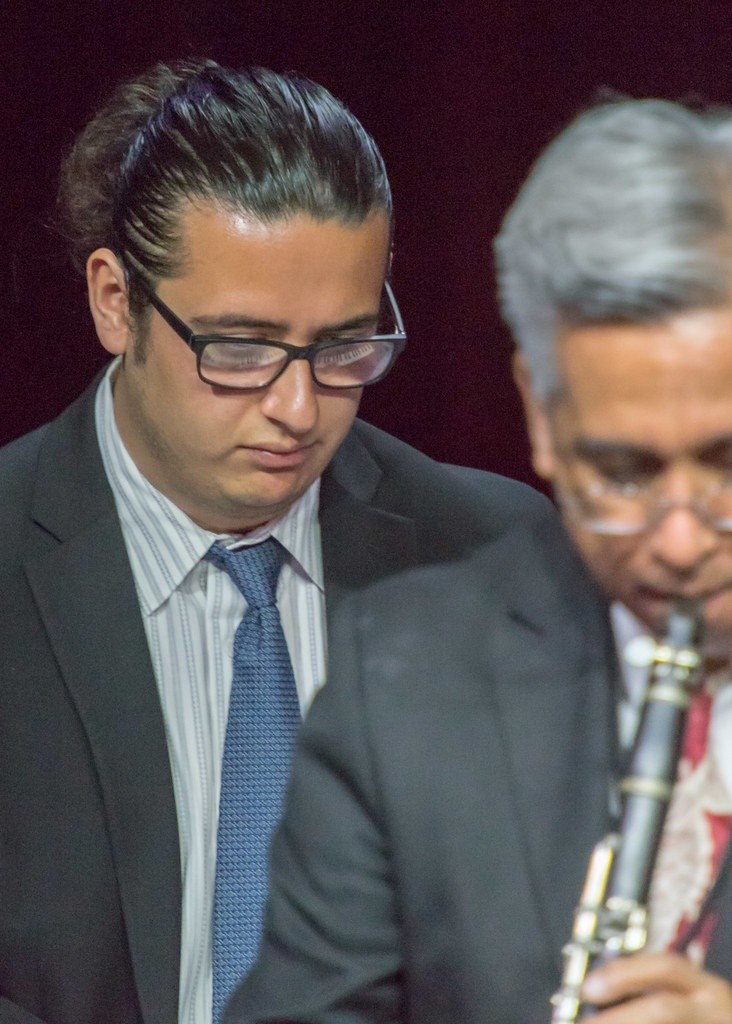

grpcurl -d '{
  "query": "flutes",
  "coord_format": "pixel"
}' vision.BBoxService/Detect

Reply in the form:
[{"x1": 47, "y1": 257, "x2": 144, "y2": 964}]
[{"x1": 544, "y1": 602, "x2": 702, "y2": 1024}]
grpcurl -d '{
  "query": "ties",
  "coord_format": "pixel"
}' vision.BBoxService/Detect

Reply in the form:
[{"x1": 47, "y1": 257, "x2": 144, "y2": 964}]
[
  {"x1": 646, "y1": 669, "x2": 732, "y2": 985},
  {"x1": 207, "y1": 536, "x2": 301, "y2": 1015}
]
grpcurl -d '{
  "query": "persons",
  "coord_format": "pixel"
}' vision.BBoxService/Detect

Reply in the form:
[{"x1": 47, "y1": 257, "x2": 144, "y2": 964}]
[
  {"x1": 220, "y1": 98, "x2": 732, "y2": 1024},
  {"x1": 0, "y1": 57, "x2": 555, "y2": 1023}
]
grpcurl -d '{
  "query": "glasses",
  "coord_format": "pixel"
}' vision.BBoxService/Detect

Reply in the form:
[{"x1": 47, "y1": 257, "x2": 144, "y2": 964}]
[
  {"x1": 106, "y1": 243, "x2": 406, "y2": 388},
  {"x1": 531, "y1": 413, "x2": 731, "y2": 510}
]
